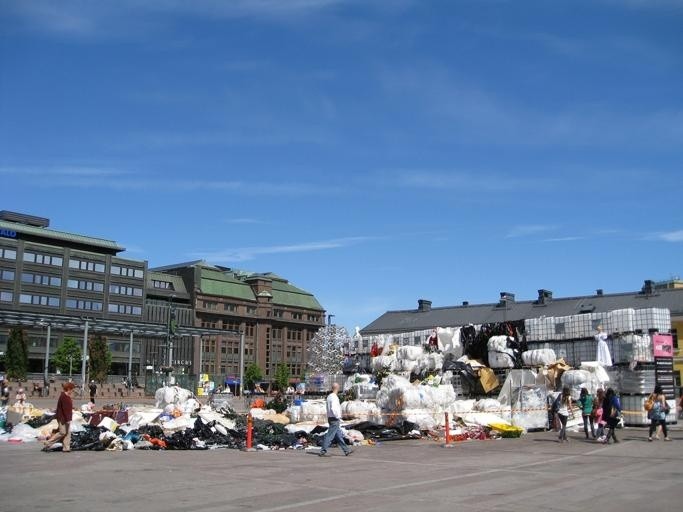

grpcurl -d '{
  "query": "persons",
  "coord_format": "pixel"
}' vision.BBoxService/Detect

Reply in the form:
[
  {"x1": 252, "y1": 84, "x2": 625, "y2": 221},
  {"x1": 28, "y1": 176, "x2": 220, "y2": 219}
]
[
  {"x1": 592, "y1": 326, "x2": 612, "y2": 368},
  {"x1": 14, "y1": 380, "x2": 26, "y2": 403},
  {"x1": 30, "y1": 377, "x2": 51, "y2": 397},
  {"x1": 646, "y1": 384, "x2": 671, "y2": 442},
  {"x1": 87, "y1": 380, "x2": 96, "y2": 404},
  {"x1": 576, "y1": 388, "x2": 596, "y2": 441},
  {"x1": 590, "y1": 388, "x2": 606, "y2": 443},
  {"x1": 601, "y1": 387, "x2": 624, "y2": 444},
  {"x1": 654, "y1": 424, "x2": 662, "y2": 440},
  {"x1": 552, "y1": 386, "x2": 575, "y2": 444},
  {"x1": 316, "y1": 382, "x2": 354, "y2": 457},
  {"x1": 0, "y1": 379, "x2": 10, "y2": 408},
  {"x1": 41, "y1": 382, "x2": 76, "y2": 454}
]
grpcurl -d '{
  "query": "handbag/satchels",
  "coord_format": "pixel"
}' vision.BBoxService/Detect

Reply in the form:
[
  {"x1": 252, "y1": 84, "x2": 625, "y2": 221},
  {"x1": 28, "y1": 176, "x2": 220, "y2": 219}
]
[
  {"x1": 645, "y1": 400, "x2": 653, "y2": 410},
  {"x1": 553, "y1": 398, "x2": 560, "y2": 411},
  {"x1": 592, "y1": 408, "x2": 602, "y2": 416}
]
